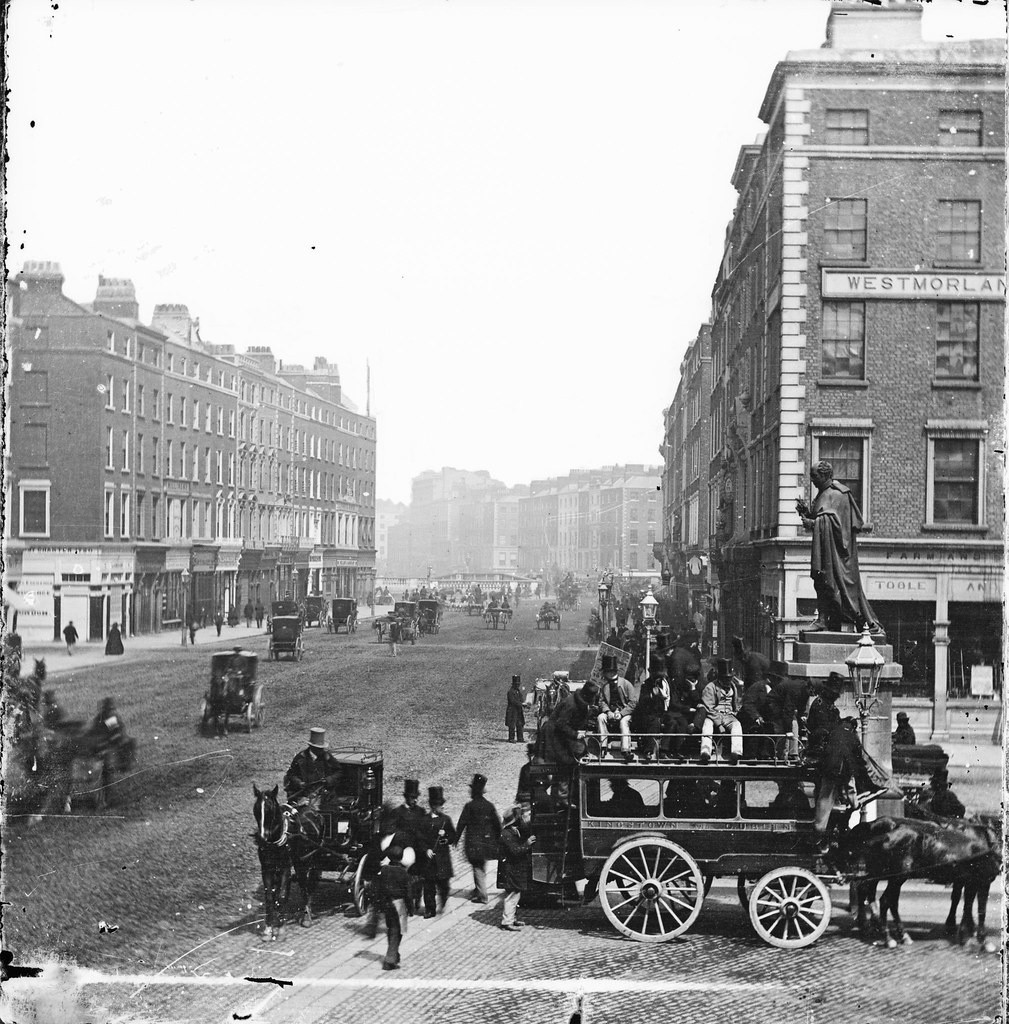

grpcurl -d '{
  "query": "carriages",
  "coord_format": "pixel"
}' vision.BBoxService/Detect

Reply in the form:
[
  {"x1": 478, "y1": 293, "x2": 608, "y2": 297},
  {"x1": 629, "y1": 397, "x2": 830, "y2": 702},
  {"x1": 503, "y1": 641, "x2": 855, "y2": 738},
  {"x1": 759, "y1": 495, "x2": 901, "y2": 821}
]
[
  {"x1": 481, "y1": 607, "x2": 510, "y2": 630},
  {"x1": 199, "y1": 649, "x2": 267, "y2": 732},
  {"x1": 536, "y1": 611, "x2": 562, "y2": 630},
  {"x1": 467, "y1": 592, "x2": 488, "y2": 616},
  {"x1": 266, "y1": 594, "x2": 359, "y2": 635},
  {"x1": 528, "y1": 708, "x2": 1005, "y2": 950},
  {"x1": 555, "y1": 586, "x2": 581, "y2": 611},
  {"x1": 1, "y1": 634, "x2": 136, "y2": 823},
  {"x1": 375, "y1": 598, "x2": 443, "y2": 646},
  {"x1": 267, "y1": 613, "x2": 307, "y2": 662},
  {"x1": 253, "y1": 744, "x2": 386, "y2": 944}
]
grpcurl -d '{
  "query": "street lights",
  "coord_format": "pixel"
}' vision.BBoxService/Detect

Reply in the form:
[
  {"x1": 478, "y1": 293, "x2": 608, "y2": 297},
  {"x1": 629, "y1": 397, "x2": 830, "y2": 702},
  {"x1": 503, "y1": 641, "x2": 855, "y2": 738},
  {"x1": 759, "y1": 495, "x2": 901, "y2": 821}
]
[
  {"x1": 598, "y1": 584, "x2": 609, "y2": 646},
  {"x1": 180, "y1": 567, "x2": 193, "y2": 649},
  {"x1": 844, "y1": 623, "x2": 887, "y2": 915},
  {"x1": 640, "y1": 584, "x2": 659, "y2": 686}
]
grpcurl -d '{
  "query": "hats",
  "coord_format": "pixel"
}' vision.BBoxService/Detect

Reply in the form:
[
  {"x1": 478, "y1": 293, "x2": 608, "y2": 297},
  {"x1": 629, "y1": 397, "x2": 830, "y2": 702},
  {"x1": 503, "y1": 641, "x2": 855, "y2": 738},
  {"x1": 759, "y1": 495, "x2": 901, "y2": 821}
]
[
  {"x1": 823, "y1": 672, "x2": 845, "y2": 695},
  {"x1": 576, "y1": 682, "x2": 598, "y2": 705},
  {"x1": 502, "y1": 805, "x2": 521, "y2": 827},
  {"x1": 429, "y1": 786, "x2": 446, "y2": 804},
  {"x1": 470, "y1": 774, "x2": 488, "y2": 793},
  {"x1": 511, "y1": 676, "x2": 523, "y2": 684},
  {"x1": 599, "y1": 656, "x2": 619, "y2": 672},
  {"x1": 306, "y1": 728, "x2": 329, "y2": 748},
  {"x1": 403, "y1": 780, "x2": 421, "y2": 796},
  {"x1": 714, "y1": 658, "x2": 736, "y2": 677},
  {"x1": 896, "y1": 712, "x2": 909, "y2": 720},
  {"x1": 765, "y1": 660, "x2": 788, "y2": 679}
]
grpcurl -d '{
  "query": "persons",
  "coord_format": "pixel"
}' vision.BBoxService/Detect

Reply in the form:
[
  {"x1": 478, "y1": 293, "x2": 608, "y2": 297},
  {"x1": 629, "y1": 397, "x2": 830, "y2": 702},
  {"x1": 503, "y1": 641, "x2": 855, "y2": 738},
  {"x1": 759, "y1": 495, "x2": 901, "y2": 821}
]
[
  {"x1": 225, "y1": 647, "x2": 248, "y2": 692},
  {"x1": 794, "y1": 462, "x2": 881, "y2": 636},
  {"x1": 536, "y1": 571, "x2": 820, "y2": 818},
  {"x1": 382, "y1": 583, "x2": 530, "y2": 657},
  {"x1": 892, "y1": 711, "x2": 916, "y2": 747},
  {"x1": 188, "y1": 608, "x2": 209, "y2": 644},
  {"x1": 283, "y1": 727, "x2": 346, "y2": 802},
  {"x1": 284, "y1": 591, "x2": 291, "y2": 601},
  {"x1": 308, "y1": 591, "x2": 315, "y2": 597},
  {"x1": 64, "y1": 621, "x2": 78, "y2": 655},
  {"x1": 928, "y1": 774, "x2": 965, "y2": 885},
  {"x1": 215, "y1": 598, "x2": 272, "y2": 637},
  {"x1": 90, "y1": 700, "x2": 124, "y2": 748},
  {"x1": 807, "y1": 671, "x2": 888, "y2": 808},
  {"x1": 454, "y1": 774, "x2": 502, "y2": 905},
  {"x1": 495, "y1": 742, "x2": 550, "y2": 932},
  {"x1": 505, "y1": 675, "x2": 527, "y2": 743},
  {"x1": 348, "y1": 780, "x2": 455, "y2": 970},
  {"x1": 105, "y1": 622, "x2": 123, "y2": 656}
]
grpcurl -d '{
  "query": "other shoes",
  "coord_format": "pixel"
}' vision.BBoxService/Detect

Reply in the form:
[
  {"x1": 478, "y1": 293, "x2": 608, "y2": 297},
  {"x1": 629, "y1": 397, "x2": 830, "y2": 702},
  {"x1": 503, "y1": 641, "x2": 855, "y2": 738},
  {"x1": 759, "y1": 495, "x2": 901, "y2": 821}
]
[
  {"x1": 730, "y1": 753, "x2": 740, "y2": 763},
  {"x1": 501, "y1": 925, "x2": 521, "y2": 932},
  {"x1": 472, "y1": 899, "x2": 488, "y2": 903},
  {"x1": 701, "y1": 753, "x2": 709, "y2": 764},
  {"x1": 858, "y1": 784, "x2": 889, "y2": 805},
  {"x1": 423, "y1": 910, "x2": 436, "y2": 918},
  {"x1": 621, "y1": 752, "x2": 633, "y2": 761},
  {"x1": 383, "y1": 962, "x2": 399, "y2": 970},
  {"x1": 397, "y1": 953, "x2": 400, "y2": 963},
  {"x1": 514, "y1": 921, "x2": 525, "y2": 926}
]
